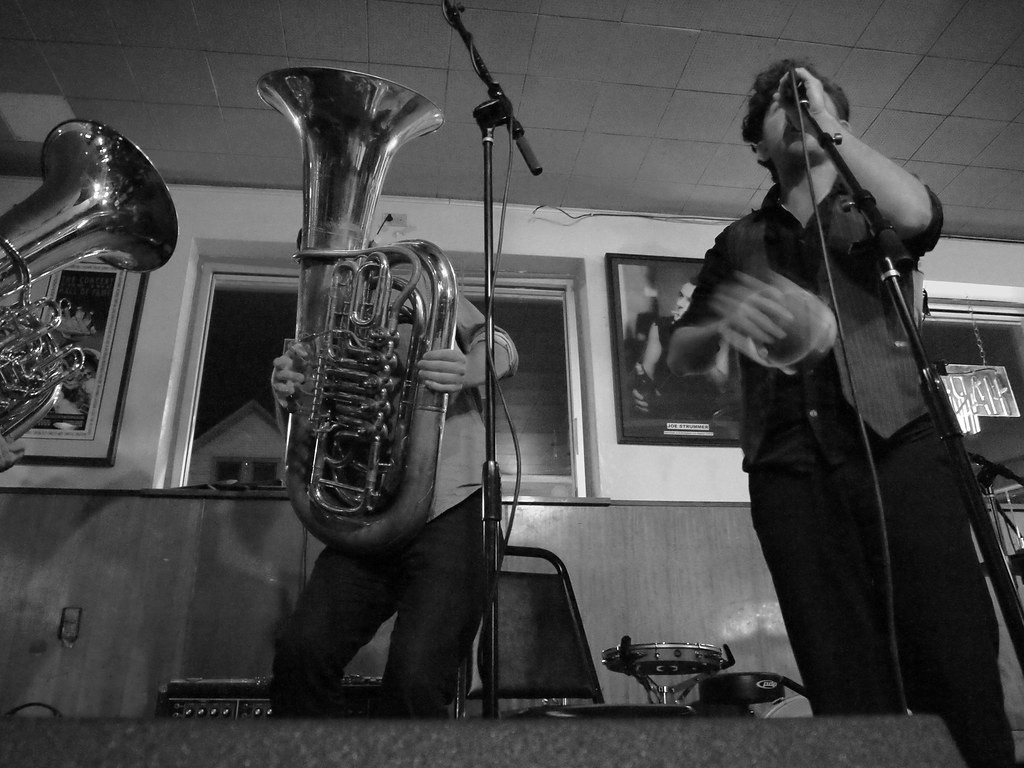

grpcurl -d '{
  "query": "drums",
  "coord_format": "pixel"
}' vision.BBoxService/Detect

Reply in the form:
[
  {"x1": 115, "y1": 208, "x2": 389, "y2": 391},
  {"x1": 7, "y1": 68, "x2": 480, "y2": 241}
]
[{"x1": 601, "y1": 641, "x2": 725, "y2": 672}]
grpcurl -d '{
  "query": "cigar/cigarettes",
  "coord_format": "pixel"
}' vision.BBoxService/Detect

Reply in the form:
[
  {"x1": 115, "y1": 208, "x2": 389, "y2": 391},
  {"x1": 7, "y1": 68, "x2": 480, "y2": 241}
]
[{"x1": 672, "y1": 311, "x2": 680, "y2": 314}]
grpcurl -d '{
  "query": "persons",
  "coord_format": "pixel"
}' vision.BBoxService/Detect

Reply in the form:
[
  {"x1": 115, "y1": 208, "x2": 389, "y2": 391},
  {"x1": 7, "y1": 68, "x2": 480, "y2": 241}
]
[
  {"x1": 626, "y1": 277, "x2": 742, "y2": 422},
  {"x1": 271, "y1": 228, "x2": 520, "y2": 719},
  {"x1": 667, "y1": 57, "x2": 1016, "y2": 767}
]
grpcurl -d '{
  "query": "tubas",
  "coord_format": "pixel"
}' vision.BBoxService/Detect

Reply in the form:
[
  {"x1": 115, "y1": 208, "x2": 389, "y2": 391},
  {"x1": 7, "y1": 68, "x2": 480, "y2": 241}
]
[
  {"x1": 0, "y1": 119, "x2": 180, "y2": 443},
  {"x1": 250, "y1": 63, "x2": 461, "y2": 557}
]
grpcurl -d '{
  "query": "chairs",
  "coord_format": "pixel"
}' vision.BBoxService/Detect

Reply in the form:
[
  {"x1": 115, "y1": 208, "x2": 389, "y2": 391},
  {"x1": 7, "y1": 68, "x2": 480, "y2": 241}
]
[{"x1": 456, "y1": 546, "x2": 695, "y2": 721}]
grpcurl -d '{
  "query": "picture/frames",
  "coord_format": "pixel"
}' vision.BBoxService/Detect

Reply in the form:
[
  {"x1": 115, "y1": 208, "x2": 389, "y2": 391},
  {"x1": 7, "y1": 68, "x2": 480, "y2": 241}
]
[
  {"x1": 14, "y1": 262, "x2": 150, "y2": 468},
  {"x1": 604, "y1": 252, "x2": 742, "y2": 448}
]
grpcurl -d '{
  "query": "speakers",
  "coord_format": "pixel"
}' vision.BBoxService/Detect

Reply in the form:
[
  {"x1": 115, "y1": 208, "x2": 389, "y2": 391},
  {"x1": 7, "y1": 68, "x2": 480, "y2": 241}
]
[{"x1": 0, "y1": 712, "x2": 965, "y2": 768}]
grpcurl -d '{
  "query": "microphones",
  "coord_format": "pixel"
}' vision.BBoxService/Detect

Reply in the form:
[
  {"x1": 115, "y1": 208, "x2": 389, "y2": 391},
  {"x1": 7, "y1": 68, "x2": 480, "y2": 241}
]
[{"x1": 785, "y1": 71, "x2": 809, "y2": 107}]
